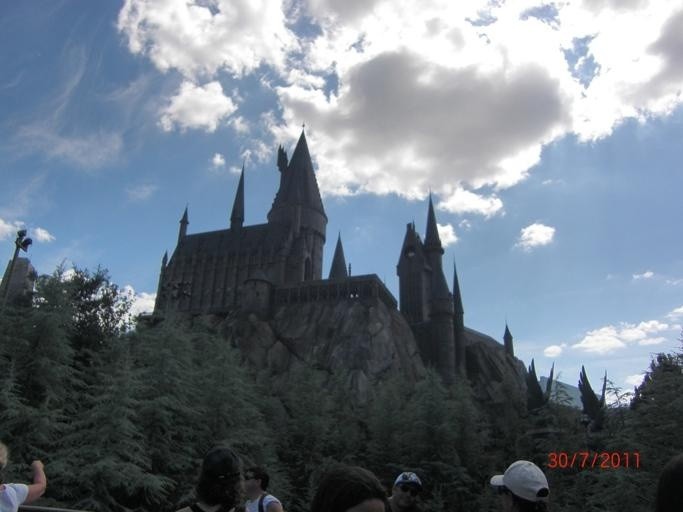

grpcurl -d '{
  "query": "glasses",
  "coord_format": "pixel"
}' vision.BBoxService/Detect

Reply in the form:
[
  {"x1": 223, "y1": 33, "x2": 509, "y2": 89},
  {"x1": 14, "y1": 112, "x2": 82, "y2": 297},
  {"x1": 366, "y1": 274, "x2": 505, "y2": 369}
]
[
  {"x1": 243, "y1": 474, "x2": 256, "y2": 481},
  {"x1": 397, "y1": 482, "x2": 419, "y2": 498}
]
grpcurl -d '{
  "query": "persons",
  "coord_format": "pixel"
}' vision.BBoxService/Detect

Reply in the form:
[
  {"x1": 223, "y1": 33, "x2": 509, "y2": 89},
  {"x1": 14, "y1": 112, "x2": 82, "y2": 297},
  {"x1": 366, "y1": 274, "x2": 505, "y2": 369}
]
[
  {"x1": 489, "y1": 459, "x2": 550, "y2": 511},
  {"x1": 175, "y1": 444, "x2": 241, "y2": 511},
  {"x1": 309, "y1": 461, "x2": 389, "y2": 511},
  {"x1": 0, "y1": 442, "x2": 46, "y2": 511},
  {"x1": 242, "y1": 465, "x2": 285, "y2": 512},
  {"x1": 385, "y1": 472, "x2": 422, "y2": 511}
]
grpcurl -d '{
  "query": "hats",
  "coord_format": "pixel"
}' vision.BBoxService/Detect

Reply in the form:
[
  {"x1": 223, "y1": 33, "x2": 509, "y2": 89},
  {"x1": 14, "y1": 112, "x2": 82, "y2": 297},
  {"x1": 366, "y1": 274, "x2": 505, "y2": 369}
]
[
  {"x1": 490, "y1": 459, "x2": 551, "y2": 502},
  {"x1": 391, "y1": 470, "x2": 422, "y2": 489}
]
